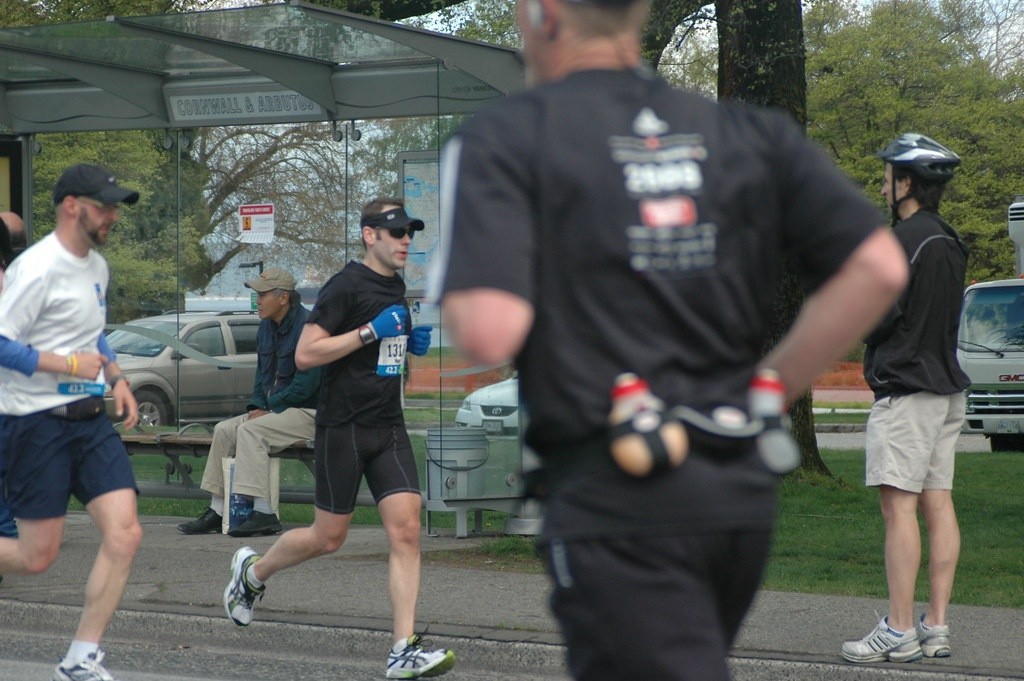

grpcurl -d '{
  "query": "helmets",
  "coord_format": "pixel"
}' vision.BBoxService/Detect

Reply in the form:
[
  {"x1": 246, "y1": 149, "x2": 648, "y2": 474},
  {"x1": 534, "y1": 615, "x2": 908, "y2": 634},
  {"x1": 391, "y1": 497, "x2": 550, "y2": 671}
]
[{"x1": 881, "y1": 132, "x2": 960, "y2": 202}]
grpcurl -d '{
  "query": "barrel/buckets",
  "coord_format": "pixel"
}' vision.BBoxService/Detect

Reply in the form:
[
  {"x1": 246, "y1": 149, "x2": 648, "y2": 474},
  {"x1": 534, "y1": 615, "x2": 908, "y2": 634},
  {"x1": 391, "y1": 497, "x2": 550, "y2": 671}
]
[{"x1": 424, "y1": 427, "x2": 490, "y2": 500}]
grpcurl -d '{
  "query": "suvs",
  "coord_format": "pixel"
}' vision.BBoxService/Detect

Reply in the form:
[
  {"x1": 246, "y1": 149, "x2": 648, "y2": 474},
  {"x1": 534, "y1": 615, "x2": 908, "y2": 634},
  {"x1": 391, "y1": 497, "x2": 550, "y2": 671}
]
[{"x1": 104, "y1": 309, "x2": 261, "y2": 425}]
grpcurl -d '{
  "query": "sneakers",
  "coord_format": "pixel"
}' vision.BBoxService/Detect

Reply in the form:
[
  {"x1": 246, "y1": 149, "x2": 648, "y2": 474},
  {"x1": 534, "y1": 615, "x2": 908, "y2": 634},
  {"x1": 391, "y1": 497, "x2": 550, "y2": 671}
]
[
  {"x1": 177, "y1": 508, "x2": 223, "y2": 534},
  {"x1": 228, "y1": 510, "x2": 284, "y2": 537},
  {"x1": 386, "y1": 624, "x2": 456, "y2": 680},
  {"x1": 916, "y1": 613, "x2": 951, "y2": 658},
  {"x1": 842, "y1": 609, "x2": 922, "y2": 663},
  {"x1": 223, "y1": 545, "x2": 266, "y2": 629},
  {"x1": 55, "y1": 647, "x2": 115, "y2": 681}
]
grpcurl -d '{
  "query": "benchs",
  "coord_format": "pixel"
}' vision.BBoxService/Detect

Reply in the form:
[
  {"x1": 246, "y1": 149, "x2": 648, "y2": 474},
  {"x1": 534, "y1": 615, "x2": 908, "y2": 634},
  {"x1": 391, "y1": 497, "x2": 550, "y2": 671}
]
[{"x1": 112, "y1": 421, "x2": 318, "y2": 491}]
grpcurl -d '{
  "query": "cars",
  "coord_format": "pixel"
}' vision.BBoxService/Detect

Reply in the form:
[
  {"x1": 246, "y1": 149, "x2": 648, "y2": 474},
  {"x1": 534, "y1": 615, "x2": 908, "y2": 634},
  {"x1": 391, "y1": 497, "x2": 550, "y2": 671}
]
[{"x1": 456, "y1": 373, "x2": 518, "y2": 436}]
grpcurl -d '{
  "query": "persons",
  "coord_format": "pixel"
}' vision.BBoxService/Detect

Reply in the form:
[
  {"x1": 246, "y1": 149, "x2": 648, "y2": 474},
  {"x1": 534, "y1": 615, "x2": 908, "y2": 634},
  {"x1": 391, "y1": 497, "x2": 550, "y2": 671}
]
[
  {"x1": 223, "y1": 195, "x2": 455, "y2": 679},
  {"x1": 436, "y1": 0, "x2": 909, "y2": 681},
  {"x1": 0, "y1": 162, "x2": 142, "y2": 680},
  {"x1": 176, "y1": 265, "x2": 316, "y2": 536},
  {"x1": 842, "y1": 130, "x2": 972, "y2": 661}
]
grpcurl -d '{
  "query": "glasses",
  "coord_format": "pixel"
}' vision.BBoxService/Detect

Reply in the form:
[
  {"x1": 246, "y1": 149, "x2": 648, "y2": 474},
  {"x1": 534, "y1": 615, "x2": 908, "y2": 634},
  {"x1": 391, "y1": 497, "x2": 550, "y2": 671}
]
[{"x1": 382, "y1": 227, "x2": 415, "y2": 239}]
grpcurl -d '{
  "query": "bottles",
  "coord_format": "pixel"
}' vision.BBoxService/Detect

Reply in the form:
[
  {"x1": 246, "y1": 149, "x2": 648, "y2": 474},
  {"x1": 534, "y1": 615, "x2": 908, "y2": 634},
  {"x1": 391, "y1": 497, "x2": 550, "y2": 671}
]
[
  {"x1": 747, "y1": 370, "x2": 801, "y2": 474},
  {"x1": 607, "y1": 372, "x2": 689, "y2": 476}
]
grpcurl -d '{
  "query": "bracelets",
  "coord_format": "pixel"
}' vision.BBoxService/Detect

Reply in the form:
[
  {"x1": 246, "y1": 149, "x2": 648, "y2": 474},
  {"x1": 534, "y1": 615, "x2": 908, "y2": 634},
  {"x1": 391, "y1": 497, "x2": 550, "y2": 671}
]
[
  {"x1": 64, "y1": 353, "x2": 78, "y2": 376},
  {"x1": 110, "y1": 373, "x2": 127, "y2": 388},
  {"x1": 359, "y1": 324, "x2": 375, "y2": 346}
]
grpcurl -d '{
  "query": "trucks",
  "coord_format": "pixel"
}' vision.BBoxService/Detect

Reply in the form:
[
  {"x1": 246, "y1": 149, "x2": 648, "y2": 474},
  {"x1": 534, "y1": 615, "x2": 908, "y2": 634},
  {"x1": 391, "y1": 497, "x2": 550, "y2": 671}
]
[{"x1": 956, "y1": 278, "x2": 1024, "y2": 451}]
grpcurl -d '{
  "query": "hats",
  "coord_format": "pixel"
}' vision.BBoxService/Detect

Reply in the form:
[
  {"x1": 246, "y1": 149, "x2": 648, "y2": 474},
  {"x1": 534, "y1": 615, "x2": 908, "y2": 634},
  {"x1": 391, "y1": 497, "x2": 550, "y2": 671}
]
[
  {"x1": 244, "y1": 266, "x2": 296, "y2": 292},
  {"x1": 54, "y1": 164, "x2": 139, "y2": 206},
  {"x1": 360, "y1": 208, "x2": 425, "y2": 231}
]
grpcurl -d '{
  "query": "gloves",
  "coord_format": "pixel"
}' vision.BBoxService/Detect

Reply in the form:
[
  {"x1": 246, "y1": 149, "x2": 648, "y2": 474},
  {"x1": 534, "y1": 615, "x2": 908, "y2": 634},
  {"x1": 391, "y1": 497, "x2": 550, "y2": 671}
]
[
  {"x1": 369, "y1": 304, "x2": 408, "y2": 340},
  {"x1": 406, "y1": 326, "x2": 432, "y2": 355}
]
